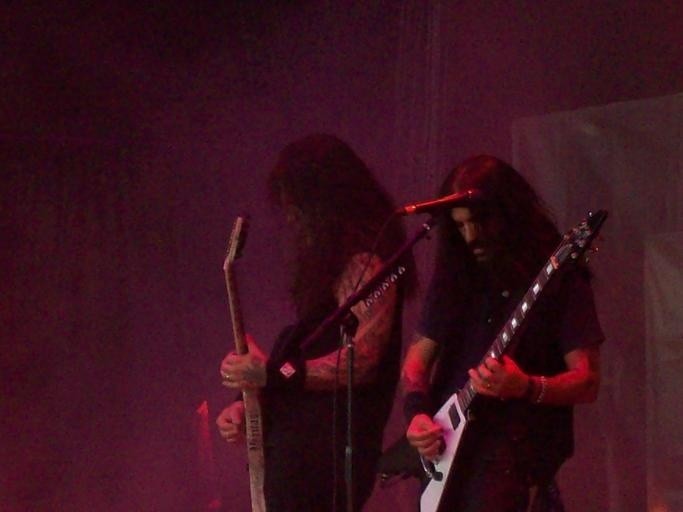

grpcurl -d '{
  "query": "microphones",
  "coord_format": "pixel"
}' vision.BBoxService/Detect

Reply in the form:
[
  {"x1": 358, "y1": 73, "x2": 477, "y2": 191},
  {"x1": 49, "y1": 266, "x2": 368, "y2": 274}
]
[{"x1": 404, "y1": 188, "x2": 483, "y2": 217}]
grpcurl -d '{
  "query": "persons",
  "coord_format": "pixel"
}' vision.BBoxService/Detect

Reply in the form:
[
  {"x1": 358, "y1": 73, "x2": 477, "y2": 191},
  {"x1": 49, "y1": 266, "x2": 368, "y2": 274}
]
[
  {"x1": 398, "y1": 158, "x2": 606, "y2": 509},
  {"x1": 213, "y1": 134, "x2": 415, "y2": 510}
]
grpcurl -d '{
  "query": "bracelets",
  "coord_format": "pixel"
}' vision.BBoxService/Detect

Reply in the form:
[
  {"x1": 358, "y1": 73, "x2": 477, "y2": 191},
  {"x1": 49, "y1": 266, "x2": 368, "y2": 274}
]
[
  {"x1": 524, "y1": 376, "x2": 534, "y2": 401},
  {"x1": 403, "y1": 392, "x2": 430, "y2": 419},
  {"x1": 536, "y1": 375, "x2": 545, "y2": 405},
  {"x1": 265, "y1": 359, "x2": 304, "y2": 393}
]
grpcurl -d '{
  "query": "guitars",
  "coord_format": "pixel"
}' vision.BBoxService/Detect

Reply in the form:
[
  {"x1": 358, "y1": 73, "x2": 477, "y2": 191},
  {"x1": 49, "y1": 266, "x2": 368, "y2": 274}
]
[
  {"x1": 370, "y1": 208, "x2": 609, "y2": 511},
  {"x1": 222, "y1": 216, "x2": 266, "y2": 511}
]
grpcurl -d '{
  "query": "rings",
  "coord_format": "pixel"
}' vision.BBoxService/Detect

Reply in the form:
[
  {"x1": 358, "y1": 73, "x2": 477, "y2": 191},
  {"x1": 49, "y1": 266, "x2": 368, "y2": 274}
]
[
  {"x1": 224, "y1": 375, "x2": 231, "y2": 380},
  {"x1": 484, "y1": 382, "x2": 491, "y2": 389}
]
